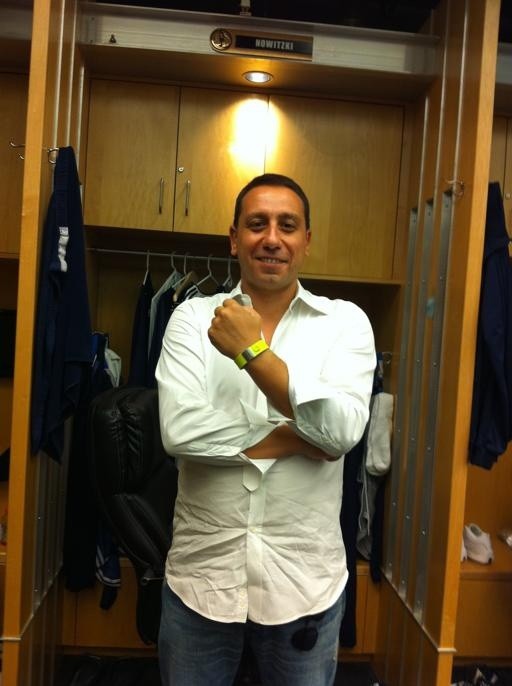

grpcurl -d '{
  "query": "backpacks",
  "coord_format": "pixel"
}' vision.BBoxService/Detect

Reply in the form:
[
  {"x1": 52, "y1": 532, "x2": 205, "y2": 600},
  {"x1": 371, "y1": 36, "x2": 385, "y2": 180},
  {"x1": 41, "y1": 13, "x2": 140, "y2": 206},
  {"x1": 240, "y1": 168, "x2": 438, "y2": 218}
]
[{"x1": 84, "y1": 385, "x2": 170, "y2": 572}]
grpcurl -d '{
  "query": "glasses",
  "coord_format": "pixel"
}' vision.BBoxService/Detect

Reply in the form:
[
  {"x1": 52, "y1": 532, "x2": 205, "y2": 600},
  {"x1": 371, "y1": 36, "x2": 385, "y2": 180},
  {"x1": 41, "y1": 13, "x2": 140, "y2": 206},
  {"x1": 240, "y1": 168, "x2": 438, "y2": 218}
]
[{"x1": 290, "y1": 613, "x2": 325, "y2": 651}]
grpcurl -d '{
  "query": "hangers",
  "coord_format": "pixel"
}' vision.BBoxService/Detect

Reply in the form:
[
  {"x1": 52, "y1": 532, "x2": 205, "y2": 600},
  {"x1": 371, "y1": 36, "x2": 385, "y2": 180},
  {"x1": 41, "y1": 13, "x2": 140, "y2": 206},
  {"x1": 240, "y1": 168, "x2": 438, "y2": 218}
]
[{"x1": 129, "y1": 246, "x2": 243, "y2": 321}]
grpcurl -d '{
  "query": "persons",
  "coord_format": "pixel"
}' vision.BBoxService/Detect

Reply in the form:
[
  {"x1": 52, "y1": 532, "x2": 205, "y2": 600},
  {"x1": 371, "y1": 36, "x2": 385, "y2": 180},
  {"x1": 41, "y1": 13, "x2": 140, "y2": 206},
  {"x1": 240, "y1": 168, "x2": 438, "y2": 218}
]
[{"x1": 156, "y1": 173, "x2": 377, "y2": 686}]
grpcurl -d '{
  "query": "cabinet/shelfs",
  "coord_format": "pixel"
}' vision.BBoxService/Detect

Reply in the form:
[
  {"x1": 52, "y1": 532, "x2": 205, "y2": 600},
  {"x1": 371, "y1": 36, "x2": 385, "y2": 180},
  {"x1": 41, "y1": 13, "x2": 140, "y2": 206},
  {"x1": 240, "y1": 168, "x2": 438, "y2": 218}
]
[
  {"x1": 265, "y1": 98, "x2": 406, "y2": 286},
  {"x1": 82, "y1": 72, "x2": 265, "y2": 245}
]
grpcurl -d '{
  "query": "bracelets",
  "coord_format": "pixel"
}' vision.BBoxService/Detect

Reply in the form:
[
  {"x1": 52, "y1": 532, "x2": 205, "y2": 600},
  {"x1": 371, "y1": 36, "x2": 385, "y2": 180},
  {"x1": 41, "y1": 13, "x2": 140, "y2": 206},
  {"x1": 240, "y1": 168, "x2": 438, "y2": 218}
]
[{"x1": 234, "y1": 339, "x2": 269, "y2": 369}]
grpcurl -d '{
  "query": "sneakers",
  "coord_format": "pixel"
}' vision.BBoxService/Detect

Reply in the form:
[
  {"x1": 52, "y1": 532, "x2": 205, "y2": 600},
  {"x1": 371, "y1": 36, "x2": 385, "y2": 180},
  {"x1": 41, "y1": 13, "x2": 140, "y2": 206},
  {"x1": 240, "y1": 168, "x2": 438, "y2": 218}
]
[
  {"x1": 461, "y1": 523, "x2": 494, "y2": 565},
  {"x1": 452, "y1": 663, "x2": 510, "y2": 686}
]
